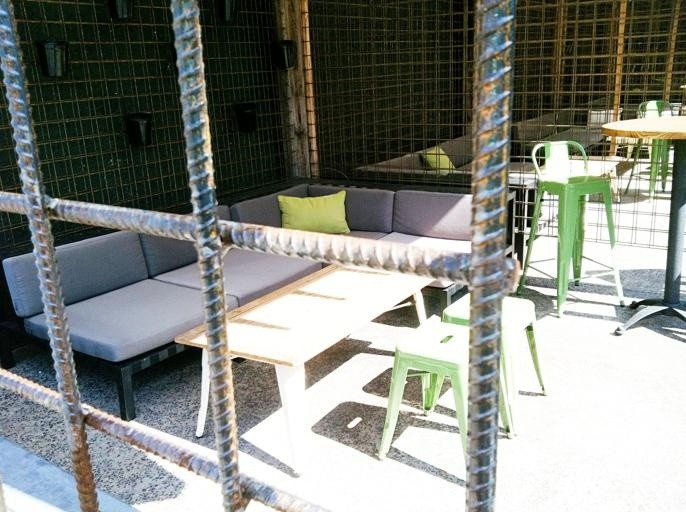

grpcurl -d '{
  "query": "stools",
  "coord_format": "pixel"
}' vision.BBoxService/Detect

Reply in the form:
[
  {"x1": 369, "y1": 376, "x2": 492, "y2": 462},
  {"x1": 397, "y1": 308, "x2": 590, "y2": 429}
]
[
  {"x1": 378, "y1": 319, "x2": 510, "y2": 460},
  {"x1": 431, "y1": 291, "x2": 547, "y2": 422}
]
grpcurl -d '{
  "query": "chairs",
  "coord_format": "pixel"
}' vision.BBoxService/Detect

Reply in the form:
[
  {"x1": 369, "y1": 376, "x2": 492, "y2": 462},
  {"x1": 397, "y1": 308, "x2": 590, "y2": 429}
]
[
  {"x1": 516, "y1": 140, "x2": 624, "y2": 318},
  {"x1": 624, "y1": 100, "x2": 675, "y2": 201}
]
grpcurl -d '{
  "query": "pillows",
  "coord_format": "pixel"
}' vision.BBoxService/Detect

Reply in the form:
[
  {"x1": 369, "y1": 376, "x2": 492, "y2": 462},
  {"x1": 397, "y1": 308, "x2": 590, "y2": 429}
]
[
  {"x1": 512, "y1": 112, "x2": 555, "y2": 144},
  {"x1": 375, "y1": 133, "x2": 473, "y2": 170},
  {"x1": 587, "y1": 107, "x2": 623, "y2": 130},
  {"x1": 557, "y1": 107, "x2": 573, "y2": 132}
]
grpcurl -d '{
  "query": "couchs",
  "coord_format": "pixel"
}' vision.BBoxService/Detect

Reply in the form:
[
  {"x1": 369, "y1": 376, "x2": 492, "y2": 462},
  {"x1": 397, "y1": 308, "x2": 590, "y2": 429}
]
[
  {"x1": 510, "y1": 108, "x2": 608, "y2": 166},
  {"x1": 0, "y1": 177, "x2": 472, "y2": 422},
  {"x1": 354, "y1": 133, "x2": 541, "y2": 227}
]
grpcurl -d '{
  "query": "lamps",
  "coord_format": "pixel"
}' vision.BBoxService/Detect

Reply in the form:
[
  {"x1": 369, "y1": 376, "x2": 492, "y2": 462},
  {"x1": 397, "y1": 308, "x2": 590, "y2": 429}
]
[
  {"x1": 36, "y1": 34, "x2": 70, "y2": 77},
  {"x1": 275, "y1": 39, "x2": 297, "y2": 68},
  {"x1": 215, "y1": 0, "x2": 238, "y2": 23},
  {"x1": 108, "y1": 0, "x2": 135, "y2": 21},
  {"x1": 232, "y1": 102, "x2": 256, "y2": 133}
]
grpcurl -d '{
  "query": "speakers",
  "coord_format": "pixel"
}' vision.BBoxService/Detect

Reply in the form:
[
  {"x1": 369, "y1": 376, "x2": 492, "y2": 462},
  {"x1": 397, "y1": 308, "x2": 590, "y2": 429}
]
[
  {"x1": 236, "y1": 103, "x2": 258, "y2": 131},
  {"x1": 269, "y1": 39, "x2": 296, "y2": 72}
]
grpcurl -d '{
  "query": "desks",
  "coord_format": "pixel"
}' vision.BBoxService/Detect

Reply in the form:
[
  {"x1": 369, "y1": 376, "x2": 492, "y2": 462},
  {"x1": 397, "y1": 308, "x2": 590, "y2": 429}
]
[{"x1": 601, "y1": 116, "x2": 686, "y2": 336}]
